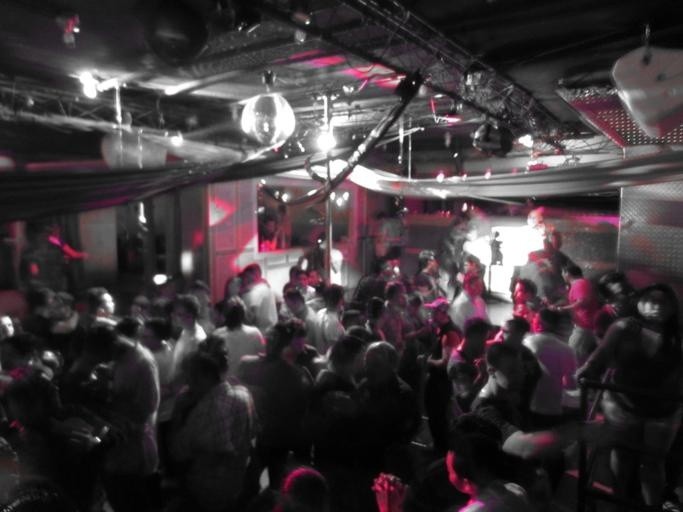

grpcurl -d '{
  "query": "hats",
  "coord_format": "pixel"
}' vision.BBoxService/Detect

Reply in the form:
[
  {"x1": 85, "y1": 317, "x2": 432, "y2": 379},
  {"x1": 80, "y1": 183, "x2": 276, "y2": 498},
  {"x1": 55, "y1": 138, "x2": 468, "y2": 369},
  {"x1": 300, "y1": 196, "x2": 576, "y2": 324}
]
[{"x1": 424, "y1": 297, "x2": 449, "y2": 312}]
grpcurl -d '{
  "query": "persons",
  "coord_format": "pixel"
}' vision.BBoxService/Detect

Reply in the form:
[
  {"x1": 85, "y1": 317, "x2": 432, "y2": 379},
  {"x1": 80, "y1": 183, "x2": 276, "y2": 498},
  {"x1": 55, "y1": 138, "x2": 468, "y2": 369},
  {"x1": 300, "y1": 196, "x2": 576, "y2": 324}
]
[{"x1": 0, "y1": 209, "x2": 682, "y2": 512}]
